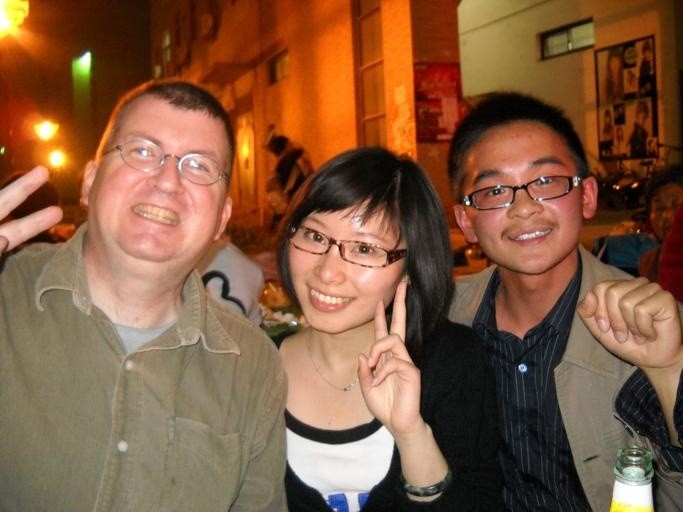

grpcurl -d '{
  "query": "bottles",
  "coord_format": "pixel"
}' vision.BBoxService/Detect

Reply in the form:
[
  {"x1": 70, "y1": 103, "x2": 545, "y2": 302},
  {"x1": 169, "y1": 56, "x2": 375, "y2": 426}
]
[{"x1": 606, "y1": 447, "x2": 656, "y2": 512}]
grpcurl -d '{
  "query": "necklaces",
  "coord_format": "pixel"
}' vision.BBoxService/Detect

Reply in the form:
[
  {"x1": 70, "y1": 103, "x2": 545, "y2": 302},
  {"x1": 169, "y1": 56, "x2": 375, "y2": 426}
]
[{"x1": 304, "y1": 324, "x2": 362, "y2": 392}]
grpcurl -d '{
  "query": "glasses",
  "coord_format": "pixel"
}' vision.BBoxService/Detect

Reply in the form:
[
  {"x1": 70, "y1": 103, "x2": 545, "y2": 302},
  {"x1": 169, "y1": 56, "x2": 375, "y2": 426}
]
[
  {"x1": 103, "y1": 139, "x2": 229, "y2": 187},
  {"x1": 285, "y1": 221, "x2": 407, "y2": 267},
  {"x1": 459, "y1": 174, "x2": 583, "y2": 211}
]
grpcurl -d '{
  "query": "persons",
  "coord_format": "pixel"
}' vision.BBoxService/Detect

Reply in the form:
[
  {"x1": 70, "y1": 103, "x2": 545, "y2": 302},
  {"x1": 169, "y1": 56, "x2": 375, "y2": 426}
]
[
  {"x1": 445, "y1": 91, "x2": 683, "y2": 512},
  {"x1": 636, "y1": 162, "x2": 683, "y2": 301},
  {"x1": 601, "y1": 40, "x2": 655, "y2": 156},
  {"x1": 197, "y1": 232, "x2": 264, "y2": 331},
  {"x1": 0, "y1": 171, "x2": 60, "y2": 243},
  {"x1": 0, "y1": 76, "x2": 289, "y2": 512},
  {"x1": 267, "y1": 144, "x2": 499, "y2": 511}
]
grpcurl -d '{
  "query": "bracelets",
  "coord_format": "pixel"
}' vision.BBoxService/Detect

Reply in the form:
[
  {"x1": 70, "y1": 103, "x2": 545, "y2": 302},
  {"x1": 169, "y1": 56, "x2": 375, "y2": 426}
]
[{"x1": 397, "y1": 468, "x2": 453, "y2": 497}]
